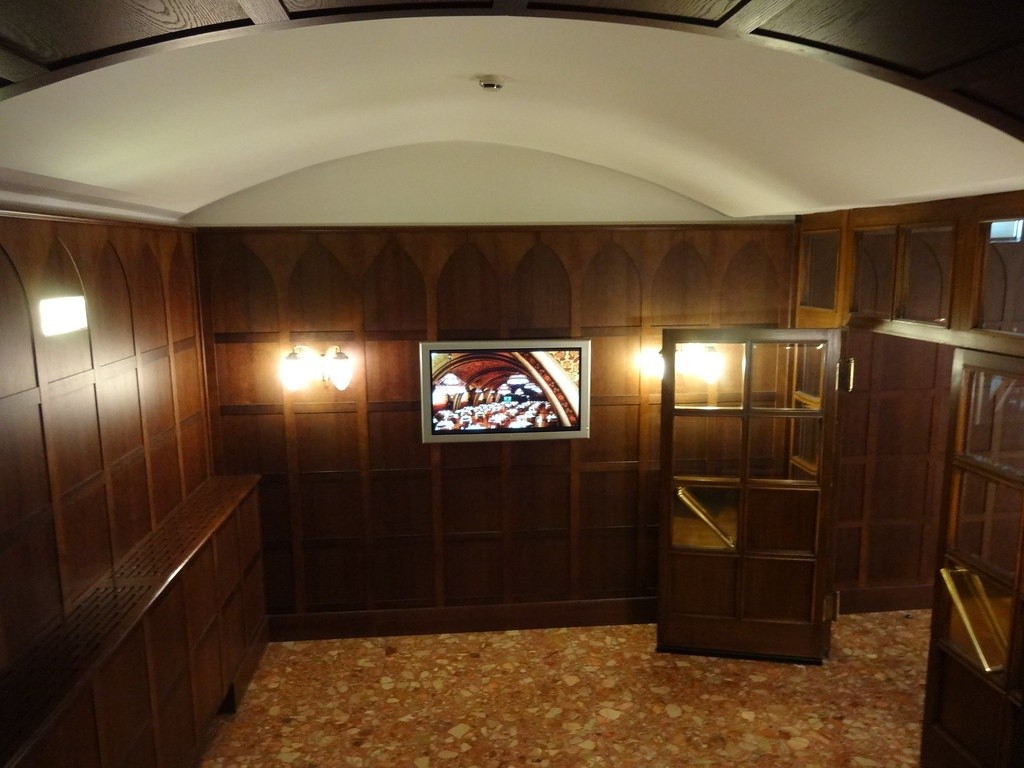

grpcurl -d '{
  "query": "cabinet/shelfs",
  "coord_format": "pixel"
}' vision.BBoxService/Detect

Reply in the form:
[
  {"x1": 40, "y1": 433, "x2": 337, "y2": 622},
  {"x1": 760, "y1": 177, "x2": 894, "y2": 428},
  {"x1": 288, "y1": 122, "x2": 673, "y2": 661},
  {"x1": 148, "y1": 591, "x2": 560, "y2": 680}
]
[{"x1": 0, "y1": 470, "x2": 268, "y2": 768}]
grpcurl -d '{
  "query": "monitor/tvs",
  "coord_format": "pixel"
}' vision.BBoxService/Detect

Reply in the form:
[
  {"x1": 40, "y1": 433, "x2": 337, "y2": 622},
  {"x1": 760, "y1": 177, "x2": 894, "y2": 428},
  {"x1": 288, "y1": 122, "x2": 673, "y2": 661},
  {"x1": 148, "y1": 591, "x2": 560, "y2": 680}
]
[{"x1": 419, "y1": 340, "x2": 591, "y2": 443}]
